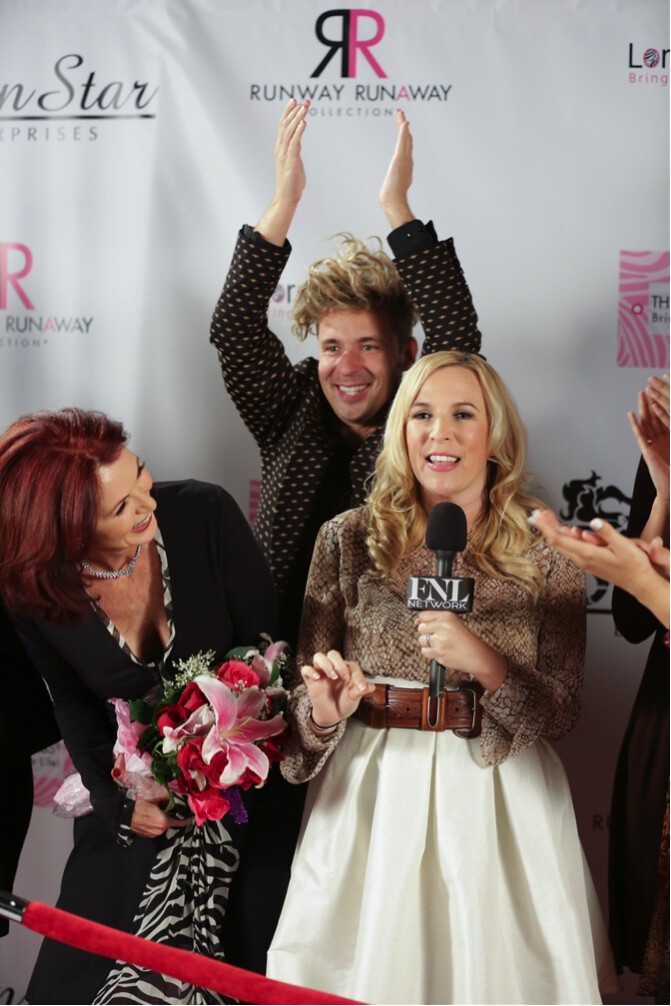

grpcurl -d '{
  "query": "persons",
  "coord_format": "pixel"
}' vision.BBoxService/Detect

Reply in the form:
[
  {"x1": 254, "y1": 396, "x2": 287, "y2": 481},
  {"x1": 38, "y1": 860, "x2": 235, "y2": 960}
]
[
  {"x1": 0, "y1": 402, "x2": 309, "y2": 1005},
  {"x1": 264, "y1": 350, "x2": 615, "y2": 1005},
  {"x1": 526, "y1": 506, "x2": 670, "y2": 647},
  {"x1": 209, "y1": 98, "x2": 482, "y2": 670},
  {"x1": 610, "y1": 368, "x2": 670, "y2": 1006}
]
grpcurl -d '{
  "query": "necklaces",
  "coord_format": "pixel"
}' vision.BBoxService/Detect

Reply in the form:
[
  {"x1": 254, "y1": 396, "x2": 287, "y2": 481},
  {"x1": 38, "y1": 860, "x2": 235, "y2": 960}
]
[{"x1": 80, "y1": 546, "x2": 141, "y2": 579}]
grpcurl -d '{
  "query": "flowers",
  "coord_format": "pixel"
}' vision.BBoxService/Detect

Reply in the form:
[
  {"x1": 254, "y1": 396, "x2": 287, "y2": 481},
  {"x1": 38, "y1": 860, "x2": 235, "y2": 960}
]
[{"x1": 55, "y1": 636, "x2": 292, "y2": 829}]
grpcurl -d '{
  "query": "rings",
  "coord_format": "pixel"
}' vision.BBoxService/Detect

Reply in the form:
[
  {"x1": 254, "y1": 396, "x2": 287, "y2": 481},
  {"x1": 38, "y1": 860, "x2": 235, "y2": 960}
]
[
  {"x1": 646, "y1": 439, "x2": 653, "y2": 444},
  {"x1": 424, "y1": 634, "x2": 432, "y2": 648}
]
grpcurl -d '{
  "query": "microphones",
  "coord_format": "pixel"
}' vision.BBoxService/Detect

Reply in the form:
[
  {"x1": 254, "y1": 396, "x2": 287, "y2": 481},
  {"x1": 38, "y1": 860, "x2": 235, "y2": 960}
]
[{"x1": 406, "y1": 501, "x2": 477, "y2": 700}]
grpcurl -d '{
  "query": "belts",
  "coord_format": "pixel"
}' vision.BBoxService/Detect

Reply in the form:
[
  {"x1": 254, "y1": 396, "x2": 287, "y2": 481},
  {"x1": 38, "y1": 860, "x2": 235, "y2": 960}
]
[{"x1": 356, "y1": 684, "x2": 488, "y2": 737}]
